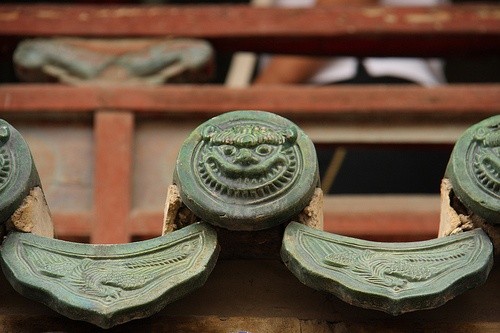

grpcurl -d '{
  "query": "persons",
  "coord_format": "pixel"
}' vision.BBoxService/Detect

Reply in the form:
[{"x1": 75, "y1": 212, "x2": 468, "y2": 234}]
[{"x1": 244, "y1": 0, "x2": 456, "y2": 194}]
